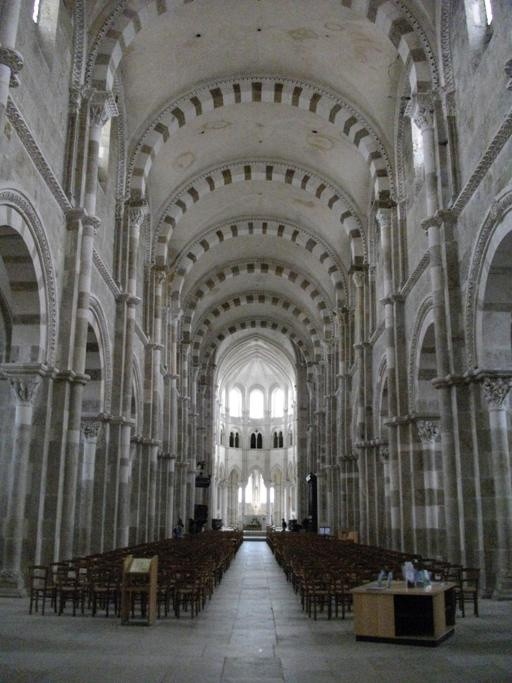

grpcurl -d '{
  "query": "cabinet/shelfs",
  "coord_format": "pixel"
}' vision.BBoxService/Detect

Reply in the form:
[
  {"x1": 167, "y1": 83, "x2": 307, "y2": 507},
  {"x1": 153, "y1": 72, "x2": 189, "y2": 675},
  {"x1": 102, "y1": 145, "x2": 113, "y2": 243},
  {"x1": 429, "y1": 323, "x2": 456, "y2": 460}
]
[{"x1": 350, "y1": 579, "x2": 456, "y2": 647}]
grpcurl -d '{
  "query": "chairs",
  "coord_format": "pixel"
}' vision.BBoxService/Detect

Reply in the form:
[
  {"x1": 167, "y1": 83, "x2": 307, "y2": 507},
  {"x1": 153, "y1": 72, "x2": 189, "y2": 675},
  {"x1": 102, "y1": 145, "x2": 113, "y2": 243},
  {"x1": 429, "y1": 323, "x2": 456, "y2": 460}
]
[
  {"x1": 264, "y1": 526, "x2": 481, "y2": 618},
  {"x1": 28, "y1": 532, "x2": 245, "y2": 618}
]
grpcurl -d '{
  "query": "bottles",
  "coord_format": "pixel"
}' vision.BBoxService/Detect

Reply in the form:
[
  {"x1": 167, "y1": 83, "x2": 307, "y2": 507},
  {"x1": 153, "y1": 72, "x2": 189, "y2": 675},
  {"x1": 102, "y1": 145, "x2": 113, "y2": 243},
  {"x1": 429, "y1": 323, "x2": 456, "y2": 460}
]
[
  {"x1": 423, "y1": 569, "x2": 432, "y2": 591},
  {"x1": 405, "y1": 561, "x2": 415, "y2": 589},
  {"x1": 376, "y1": 569, "x2": 392, "y2": 590}
]
